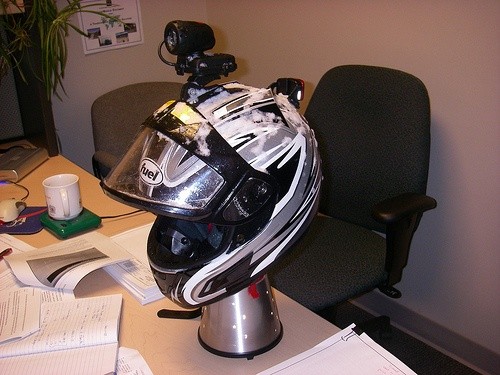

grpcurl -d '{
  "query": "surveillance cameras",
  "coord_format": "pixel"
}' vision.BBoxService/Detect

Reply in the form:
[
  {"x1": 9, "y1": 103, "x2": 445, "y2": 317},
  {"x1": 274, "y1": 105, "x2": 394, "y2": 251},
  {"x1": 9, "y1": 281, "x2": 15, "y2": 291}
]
[{"x1": 164, "y1": 19, "x2": 237, "y2": 88}]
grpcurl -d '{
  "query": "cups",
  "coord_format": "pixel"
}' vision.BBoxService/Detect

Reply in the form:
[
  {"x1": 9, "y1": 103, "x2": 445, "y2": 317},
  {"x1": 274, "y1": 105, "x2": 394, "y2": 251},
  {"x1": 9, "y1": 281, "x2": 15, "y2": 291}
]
[{"x1": 42, "y1": 174, "x2": 82, "y2": 221}]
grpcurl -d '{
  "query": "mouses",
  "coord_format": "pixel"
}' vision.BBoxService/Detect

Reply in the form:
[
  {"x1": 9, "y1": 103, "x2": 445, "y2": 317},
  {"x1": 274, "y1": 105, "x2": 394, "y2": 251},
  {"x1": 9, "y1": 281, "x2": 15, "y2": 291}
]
[{"x1": 0, "y1": 198, "x2": 26, "y2": 223}]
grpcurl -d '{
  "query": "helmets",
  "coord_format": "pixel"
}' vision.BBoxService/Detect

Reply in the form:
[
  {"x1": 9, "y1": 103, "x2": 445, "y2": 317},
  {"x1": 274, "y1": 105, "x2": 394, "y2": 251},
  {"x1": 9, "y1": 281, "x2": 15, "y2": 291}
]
[{"x1": 99, "y1": 82, "x2": 322, "y2": 309}]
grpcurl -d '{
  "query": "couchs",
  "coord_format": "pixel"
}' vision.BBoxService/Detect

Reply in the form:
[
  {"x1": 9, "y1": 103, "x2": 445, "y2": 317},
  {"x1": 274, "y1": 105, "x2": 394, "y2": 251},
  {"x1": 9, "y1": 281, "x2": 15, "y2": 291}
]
[{"x1": 90, "y1": 81, "x2": 186, "y2": 181}]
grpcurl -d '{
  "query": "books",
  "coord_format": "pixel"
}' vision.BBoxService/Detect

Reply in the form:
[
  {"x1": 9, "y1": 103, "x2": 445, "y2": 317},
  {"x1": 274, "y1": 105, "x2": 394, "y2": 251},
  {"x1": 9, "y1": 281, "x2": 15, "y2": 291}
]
[
  {"x1": 101, "y1": 222, "x2": 167, "y2": 306},
  {"x1": 0, "y1": 293, "x2": 125, "y2": 375}
]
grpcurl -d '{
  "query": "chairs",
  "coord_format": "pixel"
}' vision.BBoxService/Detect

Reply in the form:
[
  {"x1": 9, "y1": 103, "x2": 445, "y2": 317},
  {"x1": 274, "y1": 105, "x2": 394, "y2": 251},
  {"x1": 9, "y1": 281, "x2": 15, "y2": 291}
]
[{"x1": 270, "y1": 64, "x2": 438, "y2": 340}]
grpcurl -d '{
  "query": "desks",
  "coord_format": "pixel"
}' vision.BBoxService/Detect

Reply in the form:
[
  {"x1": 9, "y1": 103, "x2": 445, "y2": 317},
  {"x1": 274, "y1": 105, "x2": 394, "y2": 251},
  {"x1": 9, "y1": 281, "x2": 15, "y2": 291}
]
[{"x1": 0, "y1": 139, "x2": 418, "y2": 375}]
